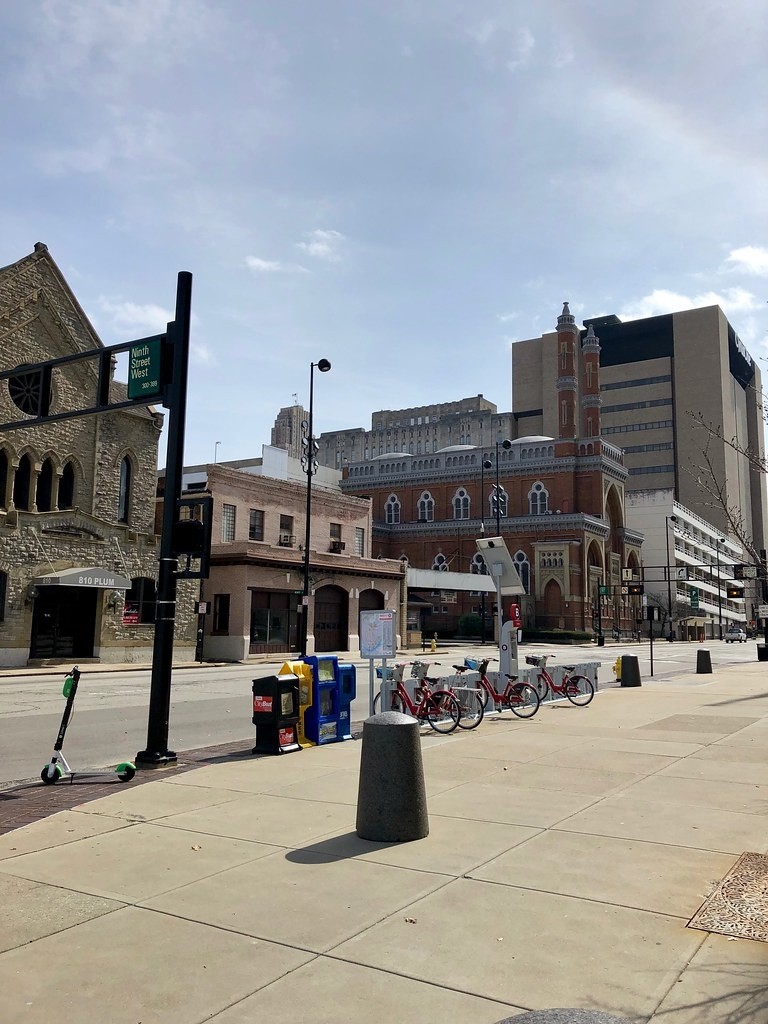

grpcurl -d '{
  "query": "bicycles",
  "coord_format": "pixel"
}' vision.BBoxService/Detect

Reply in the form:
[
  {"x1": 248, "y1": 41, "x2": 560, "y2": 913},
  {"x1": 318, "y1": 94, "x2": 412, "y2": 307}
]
[
  {"x1": 409, "y1": 661, "x2": 484, "y2": 729},
  {"x1": 524, "y1": 654, "x2": 595, "y2": 706},
  {"x1": 373, "y1": 661, "x2": 461, "y2": 734},
  {"x1": 463, "y1": 655, "x2": 540, "y2": 718}
]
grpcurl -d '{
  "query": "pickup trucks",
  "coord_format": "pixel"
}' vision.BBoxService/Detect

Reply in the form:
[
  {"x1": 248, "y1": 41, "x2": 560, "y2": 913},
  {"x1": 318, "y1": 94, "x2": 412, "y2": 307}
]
[{"x1": 725, "y1": 628, "x2": 747, "y2": 643}]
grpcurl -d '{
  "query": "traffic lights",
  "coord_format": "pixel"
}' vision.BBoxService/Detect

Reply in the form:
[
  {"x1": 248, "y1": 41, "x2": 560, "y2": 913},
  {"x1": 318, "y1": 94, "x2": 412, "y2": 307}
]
[
  {"x1": 628, "y1": 585, "x2": 644, "y2": 595},
  {"x1": 727, "y1": 588, "x2": 743, "y2": 598}
]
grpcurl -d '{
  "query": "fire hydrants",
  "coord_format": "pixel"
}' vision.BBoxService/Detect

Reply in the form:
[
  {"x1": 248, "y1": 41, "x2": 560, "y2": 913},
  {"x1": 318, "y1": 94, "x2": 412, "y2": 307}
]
[
  {"x1": 612, "y1": 655, "x2": 623, "y2": 682},
  {"x1": 431, "y1": 639, "x2": 438, "y2": 652}
]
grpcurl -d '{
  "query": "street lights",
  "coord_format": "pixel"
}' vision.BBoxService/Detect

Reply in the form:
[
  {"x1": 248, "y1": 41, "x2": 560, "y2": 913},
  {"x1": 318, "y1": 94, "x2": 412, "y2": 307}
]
[
  {"x1": 298, "y1": 356, "x2": 332, "y2": 658},
  {"x1": 716, "y1": 538, "x2": 726, "y2": 640}
]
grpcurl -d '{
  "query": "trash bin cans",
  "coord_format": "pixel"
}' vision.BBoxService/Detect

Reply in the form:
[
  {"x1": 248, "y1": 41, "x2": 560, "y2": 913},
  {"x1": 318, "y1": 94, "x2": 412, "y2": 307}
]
[{"x1": 251, "y1": 673, "x2": 303, "y2": 755}]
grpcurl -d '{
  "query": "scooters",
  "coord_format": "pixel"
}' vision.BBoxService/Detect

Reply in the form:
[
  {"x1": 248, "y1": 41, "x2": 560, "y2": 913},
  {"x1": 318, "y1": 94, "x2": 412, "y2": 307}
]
[{"x1": 41, "y1": 665, "x2": 137, "y2": 785}]
